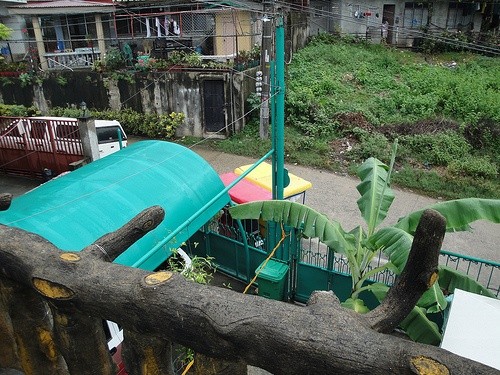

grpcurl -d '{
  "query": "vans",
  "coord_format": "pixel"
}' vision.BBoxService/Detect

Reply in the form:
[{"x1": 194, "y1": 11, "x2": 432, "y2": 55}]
[{"x1": 0, "y1": 115, "x2": 128, "y2": 160}]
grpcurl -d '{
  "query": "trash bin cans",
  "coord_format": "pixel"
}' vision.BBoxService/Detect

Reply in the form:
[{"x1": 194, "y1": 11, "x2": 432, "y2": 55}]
[{"x1": 254, "y1": 257, "x2": 291, "y2": 301}]
